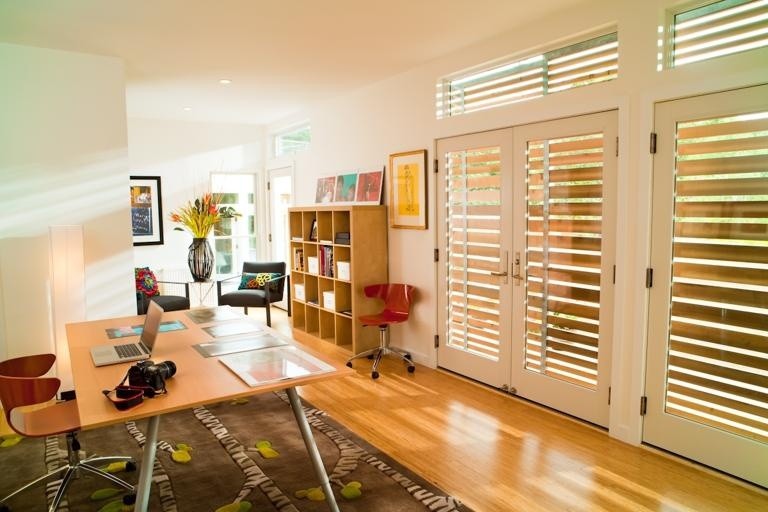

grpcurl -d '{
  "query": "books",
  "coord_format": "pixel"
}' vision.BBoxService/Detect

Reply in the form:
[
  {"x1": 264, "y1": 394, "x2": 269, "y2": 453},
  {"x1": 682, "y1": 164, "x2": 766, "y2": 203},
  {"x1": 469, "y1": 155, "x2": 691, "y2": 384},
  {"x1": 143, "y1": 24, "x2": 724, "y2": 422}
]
[{"x1": 291, "y1": 237, "x2": 334, "y2": 278}]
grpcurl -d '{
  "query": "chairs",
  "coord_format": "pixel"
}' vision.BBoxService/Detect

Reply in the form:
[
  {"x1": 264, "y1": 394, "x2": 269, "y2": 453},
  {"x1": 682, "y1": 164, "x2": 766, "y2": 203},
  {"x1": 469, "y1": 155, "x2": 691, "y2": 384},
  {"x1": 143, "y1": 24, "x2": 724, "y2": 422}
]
[
  {"x1": 134, "y1": 264, "x2": 191, "y2": 314},
  {"x1": 216, "y1": 260, "x2": 292, "y2": 328},
  {"x1": 1, "y1": 353, "x2": 140, "y2": 512},
  {"x1": 344, "y1": 281, "x2": 417, "y2": 379}
]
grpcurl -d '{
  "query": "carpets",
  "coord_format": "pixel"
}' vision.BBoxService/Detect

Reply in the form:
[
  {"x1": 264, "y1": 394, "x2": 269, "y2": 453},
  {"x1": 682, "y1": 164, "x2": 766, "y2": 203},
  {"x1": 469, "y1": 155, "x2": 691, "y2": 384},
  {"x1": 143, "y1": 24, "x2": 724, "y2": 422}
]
[{"x1": 0, "y1": 388, "x2": 477, "y2": 511}]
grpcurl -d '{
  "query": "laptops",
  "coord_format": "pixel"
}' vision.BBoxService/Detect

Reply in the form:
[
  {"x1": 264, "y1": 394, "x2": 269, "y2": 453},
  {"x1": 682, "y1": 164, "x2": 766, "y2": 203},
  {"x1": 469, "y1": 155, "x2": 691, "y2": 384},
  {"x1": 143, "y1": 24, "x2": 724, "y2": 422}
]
[{"x1": 89, "y1": 300, "x2": 164, "y2": 367}]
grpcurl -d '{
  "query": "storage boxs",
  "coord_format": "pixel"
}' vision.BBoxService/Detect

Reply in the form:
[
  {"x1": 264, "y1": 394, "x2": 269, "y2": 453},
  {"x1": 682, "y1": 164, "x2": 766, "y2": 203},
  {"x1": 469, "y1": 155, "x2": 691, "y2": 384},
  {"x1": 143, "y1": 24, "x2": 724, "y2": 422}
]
[
  {"x1": 322, "y1": 290, "x2": 336, "y2": 312},
  {"x1": 336, "y1": 260, "x2": 351, "y2": 282},
  {"x1": 307, "y1": 255, "x2": 319, "y2": 274},
  {"x1": 294, "y1": 283, "x2": 305, "y2": 302}
]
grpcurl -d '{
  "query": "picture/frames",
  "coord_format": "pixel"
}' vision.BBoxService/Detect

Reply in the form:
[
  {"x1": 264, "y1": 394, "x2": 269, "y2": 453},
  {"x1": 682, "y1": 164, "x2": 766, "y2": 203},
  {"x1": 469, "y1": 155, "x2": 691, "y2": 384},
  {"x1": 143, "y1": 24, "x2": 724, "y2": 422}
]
[
  {"x1": 356, "y1": 165, "x2": 386, "y2": 207},
  {"x1": 309, "y1": 218, "x2": 318, "y2": 242},
  {"x1": 312, "y1": 174, "x2": 338, "y2": 206},
  {"x1": 334, "y1": 170, "x2": 360, "y2": 207},
  {"x1": 389, "y1": 147, "x2": 430, "y2": 231},
  {"x1": 128, "y1": 175, "x2": 165, "y2": 247}
]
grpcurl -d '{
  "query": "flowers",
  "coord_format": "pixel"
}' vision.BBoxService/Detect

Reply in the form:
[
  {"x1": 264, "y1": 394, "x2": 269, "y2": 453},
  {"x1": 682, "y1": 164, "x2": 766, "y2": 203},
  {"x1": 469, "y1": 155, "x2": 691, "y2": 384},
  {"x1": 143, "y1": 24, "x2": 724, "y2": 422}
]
[{"x1": 166, "y1": 190, "x2": 243, "y2": 240}]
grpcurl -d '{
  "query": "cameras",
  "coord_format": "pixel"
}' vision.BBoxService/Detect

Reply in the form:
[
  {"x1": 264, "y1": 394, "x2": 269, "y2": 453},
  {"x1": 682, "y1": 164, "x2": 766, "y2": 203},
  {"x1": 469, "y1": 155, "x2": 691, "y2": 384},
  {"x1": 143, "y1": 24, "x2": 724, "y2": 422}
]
[{"x1": 128, "y1": 360, "x2": 176, "y2": 391}]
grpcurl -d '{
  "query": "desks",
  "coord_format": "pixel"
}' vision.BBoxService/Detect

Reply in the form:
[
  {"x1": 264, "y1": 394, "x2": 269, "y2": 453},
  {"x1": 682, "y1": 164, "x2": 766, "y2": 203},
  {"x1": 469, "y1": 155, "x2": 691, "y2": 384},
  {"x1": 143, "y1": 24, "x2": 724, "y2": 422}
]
[
  {"x1": 61, "y1": 303, "x2": 359, "y2": 511},
  {"x1": 156, "y1": 270, "x2": 242, "y2": 304}
]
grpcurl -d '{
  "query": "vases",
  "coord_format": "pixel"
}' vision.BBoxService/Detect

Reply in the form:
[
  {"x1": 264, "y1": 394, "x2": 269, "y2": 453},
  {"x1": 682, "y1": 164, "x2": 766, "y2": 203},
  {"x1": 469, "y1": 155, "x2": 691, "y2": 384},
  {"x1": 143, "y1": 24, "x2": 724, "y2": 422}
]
[{"x1": 186, "y1": 237, "x2": 215, "y2": 284}]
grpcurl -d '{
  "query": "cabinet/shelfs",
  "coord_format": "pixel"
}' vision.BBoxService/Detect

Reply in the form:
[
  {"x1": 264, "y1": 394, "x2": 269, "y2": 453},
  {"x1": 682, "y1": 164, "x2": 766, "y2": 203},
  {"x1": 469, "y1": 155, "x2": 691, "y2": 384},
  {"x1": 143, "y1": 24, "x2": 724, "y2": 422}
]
[{"x1": 286, "y1": 204, "x2": 388, "y2": 359}]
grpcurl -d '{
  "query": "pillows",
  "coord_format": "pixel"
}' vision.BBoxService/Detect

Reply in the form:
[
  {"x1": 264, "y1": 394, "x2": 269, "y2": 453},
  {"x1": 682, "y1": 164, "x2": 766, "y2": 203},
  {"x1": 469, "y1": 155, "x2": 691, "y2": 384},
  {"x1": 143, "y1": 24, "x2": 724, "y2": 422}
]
[
  {"x1": 134, "y1": 265, "x2": 161, "y2": 299},
  {"x1": 236, "y1": 271, "x2": 282, "y2": 290}
]
